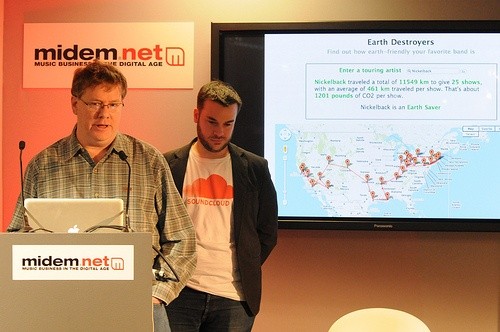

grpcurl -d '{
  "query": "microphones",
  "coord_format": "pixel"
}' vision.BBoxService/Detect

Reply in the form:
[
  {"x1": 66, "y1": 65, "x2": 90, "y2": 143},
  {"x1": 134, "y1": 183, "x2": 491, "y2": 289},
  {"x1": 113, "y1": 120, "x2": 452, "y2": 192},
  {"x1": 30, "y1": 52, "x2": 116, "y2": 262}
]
[
  {"x1": 19, "y1": 141, "x2": 35, "y2": 232},
  {"x1": 118, "y1": 150, "x2": 134, "y2": 232}
]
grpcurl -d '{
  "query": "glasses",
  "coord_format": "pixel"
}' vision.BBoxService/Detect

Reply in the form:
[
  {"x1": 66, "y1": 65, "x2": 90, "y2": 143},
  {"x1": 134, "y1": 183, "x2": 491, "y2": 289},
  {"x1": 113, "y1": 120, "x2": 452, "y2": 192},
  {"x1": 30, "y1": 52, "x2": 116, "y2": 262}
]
[{"x1": 79, "y1": 96, "x2": 124, "y2": 113}]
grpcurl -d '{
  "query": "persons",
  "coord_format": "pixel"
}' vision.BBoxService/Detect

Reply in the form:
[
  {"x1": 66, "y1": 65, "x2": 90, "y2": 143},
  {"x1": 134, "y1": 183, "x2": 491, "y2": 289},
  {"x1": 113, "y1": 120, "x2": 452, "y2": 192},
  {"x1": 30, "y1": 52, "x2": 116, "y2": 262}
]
[
  {"x1": 6, "y1": 61, "x2": 198, "y2": 332},
  {"x1": 161, "y1": 79, "x2": 279, "y2": 332}
]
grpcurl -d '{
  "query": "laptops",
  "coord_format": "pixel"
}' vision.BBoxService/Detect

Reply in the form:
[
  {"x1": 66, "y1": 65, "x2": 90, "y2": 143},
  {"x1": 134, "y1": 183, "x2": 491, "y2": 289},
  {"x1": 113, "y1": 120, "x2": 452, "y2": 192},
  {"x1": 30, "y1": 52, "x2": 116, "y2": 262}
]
[{"x1": 23, "y1": 198, "x2": 124, "y2": 232}]
